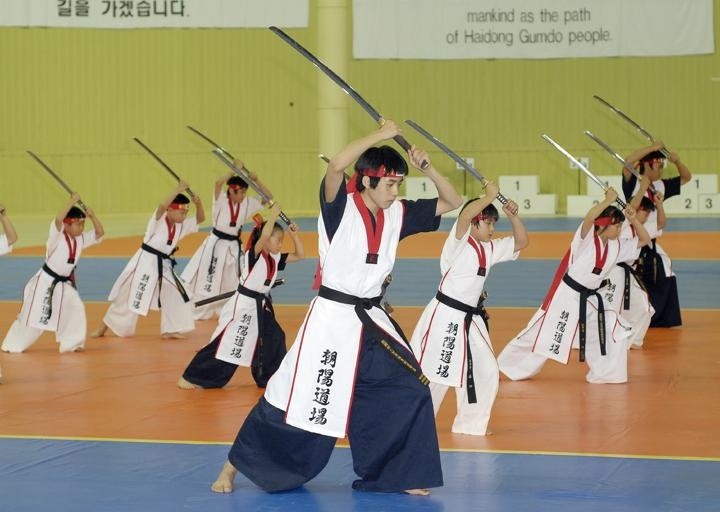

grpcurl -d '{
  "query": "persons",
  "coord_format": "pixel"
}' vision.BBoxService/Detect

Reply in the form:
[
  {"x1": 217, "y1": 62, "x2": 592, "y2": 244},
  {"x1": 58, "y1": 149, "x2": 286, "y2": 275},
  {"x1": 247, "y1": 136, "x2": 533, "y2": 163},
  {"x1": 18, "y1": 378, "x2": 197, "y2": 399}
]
[
  {"x1": 0, "y1": 191, "x2": 105, "y2": 353},
  {"x1": 623, "y1": 137, "x2": 692, "y2": 328},
  {"x1": 91, "y1": 180, "x2": 205, "y2": 339},
  {"x1": 0, "y1": 205, "x2": 18, "y2": 257},
  {"x1": 407, "y1": 177, "x2": 529, "y2": 435},
  {"x1": 571, "y1": 177, "x2": 667, "y2": 350},
  {"x1": 177, "y1": 200, "x2": 306, "y2": 391},
  {"x1": 182, "y1": 158, "x2": 274, "y2": 320},
  {"x1": 494, "y1": 183, "x2": 652, "y2": 385},
  {"x1": 210, "y1": 120, "x2": 464, "y2": 496}
]
